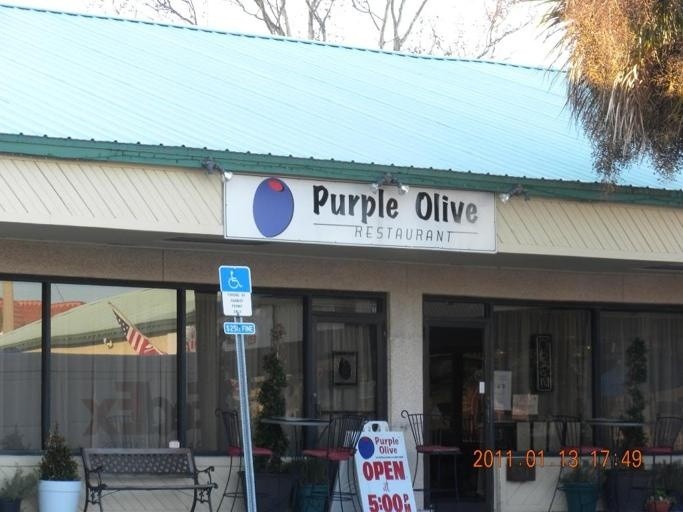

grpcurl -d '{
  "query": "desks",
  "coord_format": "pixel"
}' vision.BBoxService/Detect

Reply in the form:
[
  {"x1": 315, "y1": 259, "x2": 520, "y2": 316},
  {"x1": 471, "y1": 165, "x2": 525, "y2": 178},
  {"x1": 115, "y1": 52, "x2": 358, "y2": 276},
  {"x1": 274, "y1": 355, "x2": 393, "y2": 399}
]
[
  {"x1": 587, "y1": 417, "x2": 646, "y2": 512},
  {"x1": 510, "y1": 412, "x2": 556, "y2": 452},
  {"x1": 259, "y1": 413, "x2": 331, "y2": 510}
]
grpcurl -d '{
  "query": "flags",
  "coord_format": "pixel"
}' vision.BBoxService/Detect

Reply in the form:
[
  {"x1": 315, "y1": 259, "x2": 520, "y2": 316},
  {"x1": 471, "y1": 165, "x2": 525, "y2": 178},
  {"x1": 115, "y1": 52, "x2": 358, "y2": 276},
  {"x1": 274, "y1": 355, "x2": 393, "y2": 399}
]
[{"x1": 107, "y1": 301, "x2": 167, "y2": 356}]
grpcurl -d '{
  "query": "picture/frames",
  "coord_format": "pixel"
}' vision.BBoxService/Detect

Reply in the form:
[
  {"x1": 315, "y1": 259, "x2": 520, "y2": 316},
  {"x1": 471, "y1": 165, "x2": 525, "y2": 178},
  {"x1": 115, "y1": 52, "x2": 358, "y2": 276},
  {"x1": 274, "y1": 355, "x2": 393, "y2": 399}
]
[{"x1": 330, "y1": 348, "x2": 359, "y2": 387}]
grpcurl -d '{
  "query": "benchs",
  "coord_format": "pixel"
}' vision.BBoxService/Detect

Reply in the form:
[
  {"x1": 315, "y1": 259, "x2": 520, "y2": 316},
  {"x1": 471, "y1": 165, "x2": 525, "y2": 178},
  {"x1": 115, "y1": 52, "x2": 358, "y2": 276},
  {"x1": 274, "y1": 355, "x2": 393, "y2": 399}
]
[{"x1": 78, "y1": 444, "x2": 220, "y2": 512}]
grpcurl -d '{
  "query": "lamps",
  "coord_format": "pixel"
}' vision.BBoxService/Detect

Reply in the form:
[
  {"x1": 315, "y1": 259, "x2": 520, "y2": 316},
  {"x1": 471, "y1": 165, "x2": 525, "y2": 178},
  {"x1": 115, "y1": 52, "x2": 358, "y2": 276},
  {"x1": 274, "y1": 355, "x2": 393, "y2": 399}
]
[
  {"x1": 202, "y1": 158, "x2": 234, "y2": 187},
  {"x1": 496, "y1": 182, "x2": 529, "y2": 206},
  {"x1": 369, "y1": 172, "x2": 409, "y2": 197}
]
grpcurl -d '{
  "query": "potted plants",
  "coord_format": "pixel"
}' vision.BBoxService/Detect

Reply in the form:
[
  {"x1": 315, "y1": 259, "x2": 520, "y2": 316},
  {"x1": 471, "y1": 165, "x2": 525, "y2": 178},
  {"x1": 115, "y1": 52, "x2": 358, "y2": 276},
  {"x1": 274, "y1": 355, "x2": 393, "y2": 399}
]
[
  {"x1": 0, "y1": 461, "x2": 36, "y2": 511},
  {"x1": 554, "y1": 458, "x2": 607, "y2": 512},
  {"x1": 34, "y1": 419, "x2": 83, "y2": 511},
  {"x1": 645, "y1": 489, "x2": 677, "y2": 511}
]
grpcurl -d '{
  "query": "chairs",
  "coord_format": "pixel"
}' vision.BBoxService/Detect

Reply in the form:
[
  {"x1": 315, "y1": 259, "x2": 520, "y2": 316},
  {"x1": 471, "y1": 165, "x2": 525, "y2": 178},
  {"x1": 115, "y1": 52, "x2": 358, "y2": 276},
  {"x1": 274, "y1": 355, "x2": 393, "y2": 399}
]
[
  {"x1": 547, "y1": 411, "x2": 604, "y2": 511},
  {"x1": 637, "y1": 415, "x2": 682, "y2": 511},
  {"x1": 215, "y1": 406, "x2": 275, "y2": 511},
  {"x1": 297, "y1": 410, "x2": 368, "y2": 511},
  {"x1": 398, "y1": 407, "x2": 462, "y2": 512}
]
